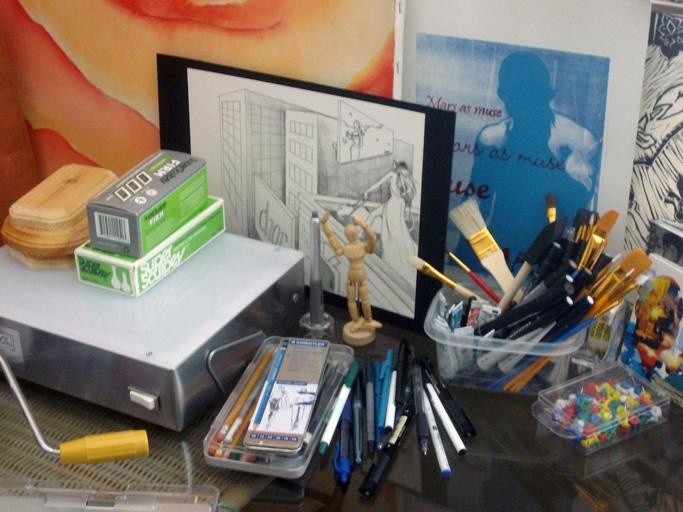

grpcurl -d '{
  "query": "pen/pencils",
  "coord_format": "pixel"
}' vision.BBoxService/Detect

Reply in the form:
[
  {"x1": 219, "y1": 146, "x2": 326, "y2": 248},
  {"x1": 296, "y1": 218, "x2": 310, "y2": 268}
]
[
  {"x1": 201, "y1": 339, "x2": 474, "y2": 505},
  {"x1": 475, "y1": 208, "x2": 596, "y2": 388}
]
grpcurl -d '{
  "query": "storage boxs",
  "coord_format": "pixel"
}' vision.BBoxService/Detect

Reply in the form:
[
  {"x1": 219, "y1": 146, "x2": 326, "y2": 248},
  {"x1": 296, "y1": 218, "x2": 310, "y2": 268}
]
[{"x1": 424, "y1": 282, "x2": 587, "y2": 395}]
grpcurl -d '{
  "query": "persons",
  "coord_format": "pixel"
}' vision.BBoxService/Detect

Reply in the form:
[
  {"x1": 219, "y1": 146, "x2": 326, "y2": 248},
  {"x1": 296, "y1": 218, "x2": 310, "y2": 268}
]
[
  {"x1": 451, "y1": 50, "x2": 601, "y2": 278},
  {"x1": 359, "y1": 160, "x2": 420, "y2": 269},
  {"x1": 319, "y1": 212, "x2": 387, "y2": 332}
]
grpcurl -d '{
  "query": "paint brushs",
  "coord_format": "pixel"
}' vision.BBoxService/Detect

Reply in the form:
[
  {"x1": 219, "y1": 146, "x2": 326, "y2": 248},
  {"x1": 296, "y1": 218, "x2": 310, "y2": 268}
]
[
  {"x1": 405, "y1": 193, "x2": 556, "y2": 304},
  {"x1": 487, "y1": 210, "x2": 652, "y2": 392}
]
